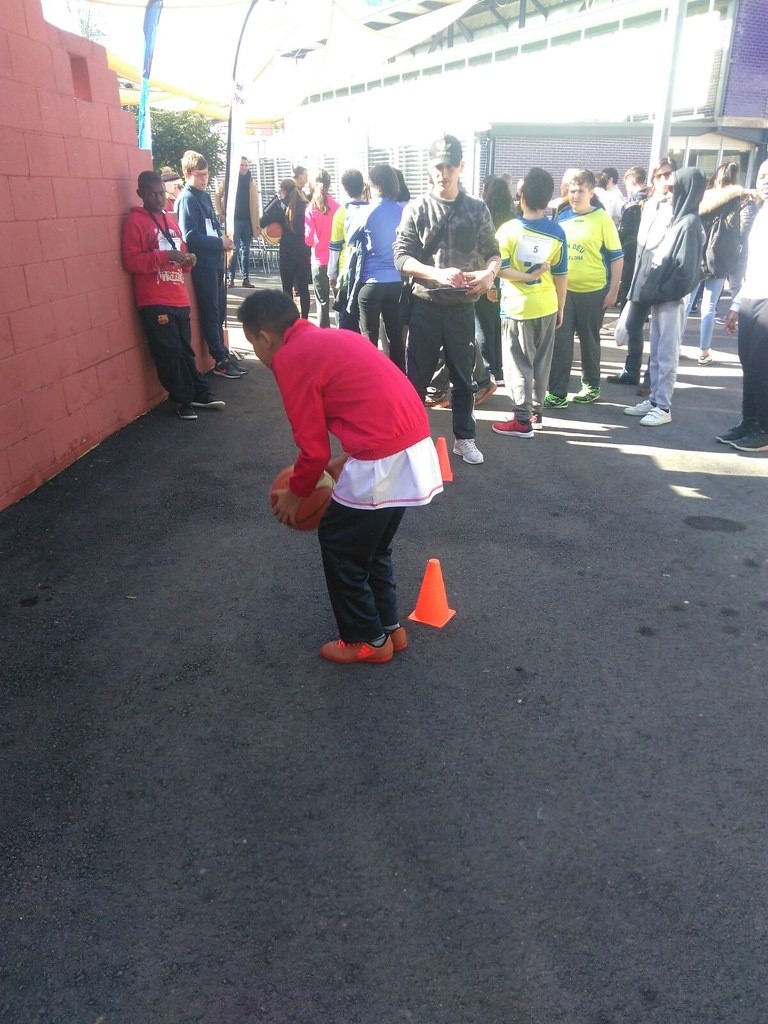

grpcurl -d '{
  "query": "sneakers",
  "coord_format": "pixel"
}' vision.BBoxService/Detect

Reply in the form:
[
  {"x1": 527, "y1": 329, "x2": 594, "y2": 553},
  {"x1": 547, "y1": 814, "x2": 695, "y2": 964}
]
[
  {"x1": 531, "y1": 415, "x2": 543, "y2": 429},
  {"x1": 171, "y1": 395, "x2": 198, "y2": 419},
  {"x1": 730, "y1": 428, "x2": 768, "y2": 451},
  {"x1": 636, "y1": 385, "x2": 650, "y2": 397},
  {"x1": 212, "y1": 362, "x2": 240, "y2": 379},
  {"x1": 640, "y1": 407, "x2": 672, "y2": 426},
  {"x1": 543, "y1": 395, "x2": 569, "y2": 409},
  {"x1": 573, "y1": 386, "x2": 601, "y2": 404},
  {"x1": 391, "y1": 627, "x2": 407, "y2": 651},
  {"x1": 492, "y1": 418, "x2": 535, "y2": 438},
  {"x1": 623, "y1": 399, "x2": 654, "y2": 416},
  {"x1": 606, "y1": 373, "x2": 640, "y2": 385},
  {"x1": 227, "y1": 280, "x2": 235, "y2": 288},
  {"x1": 242, "y1": 280, "x2": 255, "y2": 288},
  {"x1": 320, "y1": 634, "x2": 394, "y2": 664},
  {"x1": 699, "y1": 354, "x2": 712, "y2": 366},
  {"x1": 453, "y1": 438, "x2": 484, "y2": 464},
  {"x1": 716, "y1": 419, "x2": 757, "y2": 444},
  {"x1": 229, "y1": 357, "x2": 248, "y2": 374},
  {"x1": 191, "y1": 391, "x2": 225, "y2": 409}
]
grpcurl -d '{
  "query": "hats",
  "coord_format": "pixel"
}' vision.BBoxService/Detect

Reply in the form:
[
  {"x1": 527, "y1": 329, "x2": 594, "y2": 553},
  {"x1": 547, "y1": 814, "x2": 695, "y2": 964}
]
[{"x1": 426, "y1": 134, "x2": 462, "y2": 167}]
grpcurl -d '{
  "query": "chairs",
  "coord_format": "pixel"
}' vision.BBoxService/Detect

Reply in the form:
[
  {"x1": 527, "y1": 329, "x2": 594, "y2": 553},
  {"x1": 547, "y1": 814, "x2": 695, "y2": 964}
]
[{"x1": 236, "y1": 234, "x2": 280, "y2": 274}]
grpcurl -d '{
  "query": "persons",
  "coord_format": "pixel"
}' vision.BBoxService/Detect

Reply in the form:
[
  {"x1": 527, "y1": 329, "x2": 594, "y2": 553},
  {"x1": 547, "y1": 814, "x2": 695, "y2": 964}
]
[
  {"x1": 624, "y1": 166, "x2": 706, "y2": 427},
  {"x1": 558, "y1": 167, "x2": 649, "y2": 317},
  {"x1": 543, "y1": 168, "x2": 625, "y2": 408},
  {"x1": 259, "y1": 164, "x2": 413, "y2": 375},
  {"x1": 679, "y1": 162, "x2": 744, "y2": 368},
  {"x1": 174, "y1": 151, "x2": 249, "y2": 378},
  {"x1": 121, "y1": 170, "x2": 226, "y2": 418},
  {"x1": 715, "y1": 188, "x2": 759, "y2": 326},
  {"x1": 608, "y1": 156, "x2": 677, "y2": 396},
  {"x1": 236, "y1": 288, "x2": 445, "y2": 664},
  {"x1": 160, "y1": 167, "x2": 183, "y2": 212},
  {"x1": 715, "y1": 159, "x2": 768, "y2": 450},
  {"x1": 214, "y1": 156, "x2": 260, "y2": 288},
  {"x1": 492, "y1": 167, "x2": 569, "y2": 438},
  {"x1": 392, "y1": 133, "x2": 518, "y2": 464}
]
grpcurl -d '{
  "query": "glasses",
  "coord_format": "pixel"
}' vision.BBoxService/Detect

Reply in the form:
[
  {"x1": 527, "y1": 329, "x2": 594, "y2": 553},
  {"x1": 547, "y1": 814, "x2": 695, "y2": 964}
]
[
  {"x1": 191, "y1": 172, "x2": 209, "y2": 178},
  {"x1": 655, "y1": 171, "x2": 670, "y2": 179}
]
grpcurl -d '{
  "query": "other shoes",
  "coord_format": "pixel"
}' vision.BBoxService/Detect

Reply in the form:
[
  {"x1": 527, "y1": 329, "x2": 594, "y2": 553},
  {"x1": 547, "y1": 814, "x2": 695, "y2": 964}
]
[
  {"x1": 473, "y1": 383, "x2": 497, "y2": 407},
  {"x1": 425, "y1": 394, "x2": 449, "y2": 408}
]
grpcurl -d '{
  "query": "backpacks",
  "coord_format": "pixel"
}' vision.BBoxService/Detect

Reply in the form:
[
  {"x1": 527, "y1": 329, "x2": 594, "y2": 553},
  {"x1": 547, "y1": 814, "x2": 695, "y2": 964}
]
[{"x1": 705, "y1": 208, "x2": 737, "y2": 277}]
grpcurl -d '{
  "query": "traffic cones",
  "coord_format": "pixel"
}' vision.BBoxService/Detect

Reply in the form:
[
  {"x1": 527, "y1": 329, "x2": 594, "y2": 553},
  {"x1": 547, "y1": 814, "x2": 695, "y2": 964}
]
[
  {"x1": 407, "y1": 557, "x2": 457, "y2": 628},
  {"x1": 435, "y1": 436, "x2": 454, "y2": 483}
]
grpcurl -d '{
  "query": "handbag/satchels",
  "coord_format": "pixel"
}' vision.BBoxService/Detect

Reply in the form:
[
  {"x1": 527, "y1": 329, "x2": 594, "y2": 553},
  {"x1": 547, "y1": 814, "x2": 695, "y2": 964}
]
[{"x1": 614, "y1": 300, "x2": 633, "y2": 346}]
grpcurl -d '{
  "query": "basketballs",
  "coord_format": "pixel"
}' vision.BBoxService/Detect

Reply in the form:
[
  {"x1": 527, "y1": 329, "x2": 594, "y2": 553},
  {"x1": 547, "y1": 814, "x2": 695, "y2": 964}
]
[
  {"x1": 261, "y1": 222, "x2": 282, "y2": 245},
  {"x1": 270, "y1": 463, "x2": 336, "y2": 532}
]
